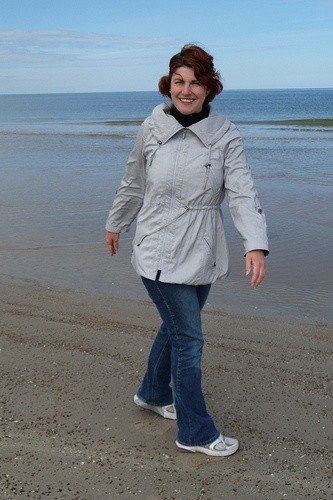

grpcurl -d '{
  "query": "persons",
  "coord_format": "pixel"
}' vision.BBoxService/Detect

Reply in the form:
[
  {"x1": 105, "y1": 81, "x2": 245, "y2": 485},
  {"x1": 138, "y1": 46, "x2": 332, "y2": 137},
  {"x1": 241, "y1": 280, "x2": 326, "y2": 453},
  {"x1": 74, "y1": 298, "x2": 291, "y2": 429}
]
[{"x1": 104, "y1": 43, "x2": 270, "y2": 457}]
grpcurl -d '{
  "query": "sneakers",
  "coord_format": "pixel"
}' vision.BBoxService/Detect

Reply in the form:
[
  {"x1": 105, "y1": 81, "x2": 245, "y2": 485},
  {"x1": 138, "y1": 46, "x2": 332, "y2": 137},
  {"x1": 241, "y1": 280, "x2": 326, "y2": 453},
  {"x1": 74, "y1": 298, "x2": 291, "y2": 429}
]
[
  {"x1": 133, "y1": 394, "x2": 178, "y2": 420},
  {"x1": 175, "y1": 433, "x2": 239, "y2": 457}
]
